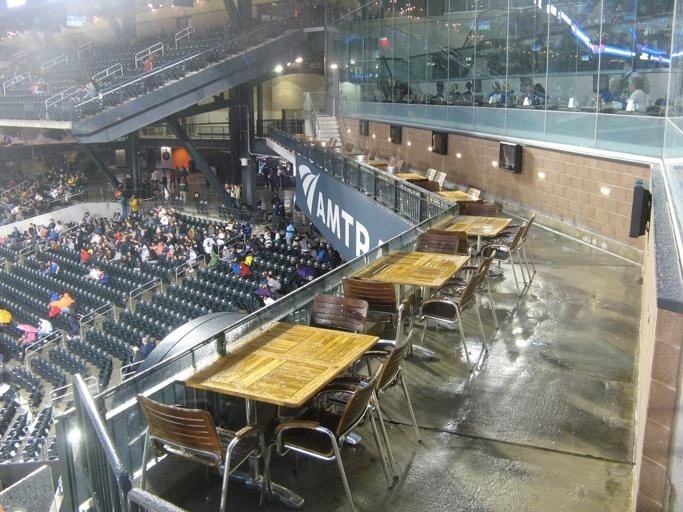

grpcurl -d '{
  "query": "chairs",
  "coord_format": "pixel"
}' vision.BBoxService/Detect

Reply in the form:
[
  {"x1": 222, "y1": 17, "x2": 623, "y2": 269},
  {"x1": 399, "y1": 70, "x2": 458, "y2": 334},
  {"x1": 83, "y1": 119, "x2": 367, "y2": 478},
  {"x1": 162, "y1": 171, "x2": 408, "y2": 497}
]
[
  {"x1": 418, "y1": 263, "x2": 491, "y2": 372},
  {"x1": 465, "y1": 203, "x2": 497, "y2": 217},
  {"x1": 137, "y1": 391, "x2": 272, "y2": 512},
  {"x1": 274, "y1": 363, "x2": 391, "y2": 508},
  {"x1": 460, "y1": 250, "x2": 501, "y2": 332},
  {"x1": 498, "y1": 208, "x2": 536, "y2": 284},
  {"x1": 342, "y1": 274, "x2": 416, "y2": 365},
  {"x1": 428, "y1": 227, "x2": 471, "y2": 256},
  {"x1": 328, "y1": 325, "x2": 422, "y2": 483},
  {"x1": 292, "y1": 133, "x2": 484, "y2": 201},
  {"x1": 415, "y1": 232, "x2": 459, "y2": 256},
  {"x1": 475, "y1": 219, "x2": 531, "y2": 298},
  {"x1": 2, "y1": 211, "x2": 352, "y2": 466},
  {"x1": 309, "y1": 291, "x2": 375, "y2": 380}
]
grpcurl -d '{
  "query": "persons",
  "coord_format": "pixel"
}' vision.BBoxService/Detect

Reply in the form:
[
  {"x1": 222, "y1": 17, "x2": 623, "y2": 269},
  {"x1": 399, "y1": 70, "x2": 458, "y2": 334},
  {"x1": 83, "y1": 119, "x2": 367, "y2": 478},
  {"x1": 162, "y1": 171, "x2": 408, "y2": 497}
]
[{"x1": 0, "y1": 0, "x2": 648, "y2": 371}]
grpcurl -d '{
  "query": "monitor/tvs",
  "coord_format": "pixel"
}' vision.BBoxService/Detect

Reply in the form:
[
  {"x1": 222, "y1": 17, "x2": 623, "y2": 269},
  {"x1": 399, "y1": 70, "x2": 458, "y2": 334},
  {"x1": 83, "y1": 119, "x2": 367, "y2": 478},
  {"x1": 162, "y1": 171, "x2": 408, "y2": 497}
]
[
  {"x1": 520, "y1": 77, "x2": 530, "y2": 92},
  {"x1": 432, "y1": 132, "x2": 447, "y2": 155},
  {"x1": 628, "y1": 178, "x2": 651, "y2": 238},
  {"x1": 390, "y1": 125, "x2": 402, "y2": 144},
  {"x1": 499, "y1": 141, "x2": 522, "y2": 172},
  {"x1": 593, "y1": 74, "x2": 608, "y2": 92},
  {"x1": 359, "y1": 119, "x2": 369, "y2": 135},
  {"x1": 437, "y1": 80, "x2": 443, "y2": 92},
  {"x1": 472, "y1": 79, "x2": 481, "y2": 92}
]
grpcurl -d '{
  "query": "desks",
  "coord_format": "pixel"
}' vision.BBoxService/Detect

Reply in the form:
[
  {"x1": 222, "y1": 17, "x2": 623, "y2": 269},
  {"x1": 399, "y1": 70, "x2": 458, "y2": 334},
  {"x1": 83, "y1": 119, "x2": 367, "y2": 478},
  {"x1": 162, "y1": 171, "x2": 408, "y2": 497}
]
[
  {"x1": 427, "y1": 215, "x2": 514, "y2": 278},
  {"x1": 347, "y1": 249, "x2": 469, "y2": 362},
  {"x1": 185, "y1": 320, "x2": 380, "y2": 510}
]
